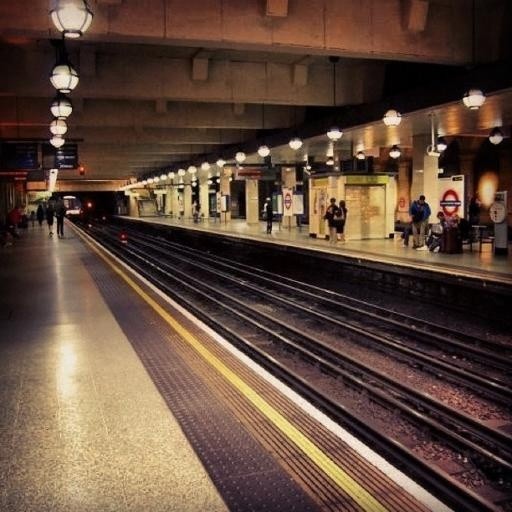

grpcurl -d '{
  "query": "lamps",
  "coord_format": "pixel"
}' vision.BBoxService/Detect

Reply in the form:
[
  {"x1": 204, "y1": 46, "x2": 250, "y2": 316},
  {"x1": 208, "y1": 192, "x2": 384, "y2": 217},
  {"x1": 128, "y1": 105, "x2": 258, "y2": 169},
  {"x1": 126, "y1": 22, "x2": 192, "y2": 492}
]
[
  {"x1": 461, "y1": 1, "x2": 488, "y2": 111},
  {"x1": 256, "y1": 102, "x2": 272, "y2": 157},
  {"x1": 140, "y1": 125, "x2": 247, "y2": 186},
  {"x1": 287, "y1": 106, "x2": 304, "y2": 150},
  {"x1": 323, "y1": 125, "x2": 504, "y2": 166},
  {"x1": 324, "y1": 56, "x2": 343, "y2": 143},
  {"x1": 381, "y1": 103, "x2": 403, "y2": 128},
  {"x1": 45, "y1": 1, "x2": 96, "y2": 149}
]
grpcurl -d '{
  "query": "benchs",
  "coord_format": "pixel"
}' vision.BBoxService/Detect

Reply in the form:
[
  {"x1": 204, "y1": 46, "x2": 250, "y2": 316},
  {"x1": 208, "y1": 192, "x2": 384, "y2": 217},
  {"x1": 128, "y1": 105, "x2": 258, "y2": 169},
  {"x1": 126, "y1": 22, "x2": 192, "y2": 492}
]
[{"x1": 393, "y1": 219, "x2": 494, "y2": 254}]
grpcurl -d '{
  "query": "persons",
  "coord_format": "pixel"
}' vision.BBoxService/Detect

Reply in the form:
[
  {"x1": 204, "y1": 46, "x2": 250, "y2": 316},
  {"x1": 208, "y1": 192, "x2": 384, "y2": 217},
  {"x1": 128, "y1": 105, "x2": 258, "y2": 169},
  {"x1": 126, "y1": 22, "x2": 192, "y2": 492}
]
[
  {"x1": 45, "y1": 204, "x2": 54, "y2": 237},
  {"x1": 37, "y1": 205, "x2": 44, "y2": 225},
  {"x1": 337, "y1": 200, "x2": 348, "y2": 244},
  {"x1": 410, "y1": 195, "x2": 431, "y2": 248},
  {"x1": 323, "y1": 198, "x2": 338, "y2": 248},
  {"x1": 468, "y1": 192, "x2": 481, "y2": 225},
  {"x1": 264, "y1": 197, "x2": 274, "y2": 233},
  {"x1": 55, "y1": 196, "x2": 66, "y2": 238},
  {"x1": 428, "y1": 212, "x2": 470, "y2": 252}
]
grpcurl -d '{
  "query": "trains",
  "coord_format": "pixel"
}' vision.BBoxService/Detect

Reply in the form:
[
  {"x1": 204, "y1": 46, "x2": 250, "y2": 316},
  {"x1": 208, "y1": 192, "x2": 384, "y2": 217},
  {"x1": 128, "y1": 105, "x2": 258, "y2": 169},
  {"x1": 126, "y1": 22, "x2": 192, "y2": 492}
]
[{"x1": 62, "y1": 195, "x2": 83, "y2": 222}]
